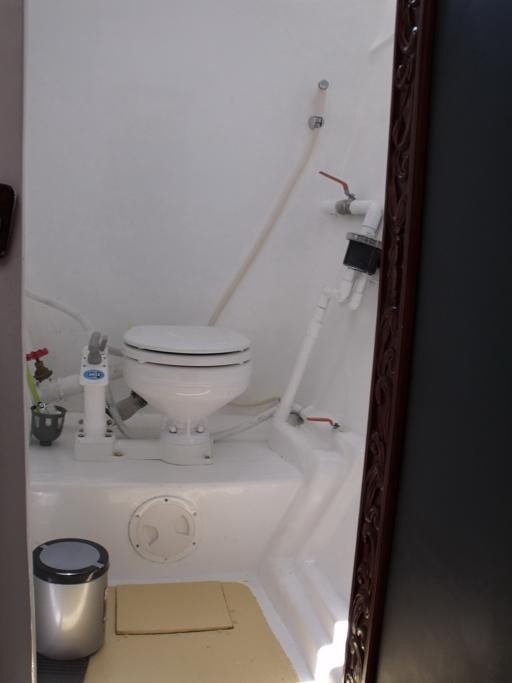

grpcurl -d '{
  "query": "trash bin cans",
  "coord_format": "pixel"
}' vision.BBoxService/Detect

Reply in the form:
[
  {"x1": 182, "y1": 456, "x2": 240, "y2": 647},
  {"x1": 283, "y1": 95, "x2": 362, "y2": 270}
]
[{"x1": 33, "y1": 537, "x2": 108, "y2": 661}]
